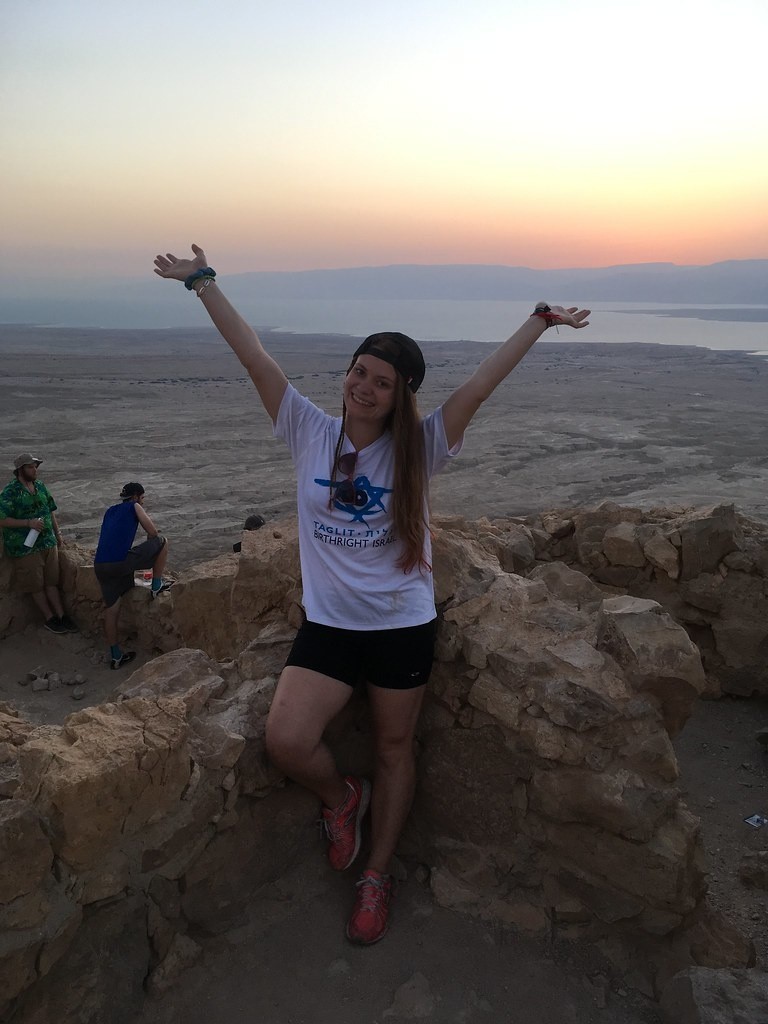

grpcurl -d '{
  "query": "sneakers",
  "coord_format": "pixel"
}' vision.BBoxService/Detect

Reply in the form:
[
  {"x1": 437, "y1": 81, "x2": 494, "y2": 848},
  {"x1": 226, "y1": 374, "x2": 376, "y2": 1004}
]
[
  {"x1": 43, "y1": 614, "x2": 80, "y2": 634},
  {"x1": 110, "y1": 652, "x2": 136, "y2": 670},
  {"x1": 346, "y1": 869, "x2": 399, "y2": 946},
  {"x1": 314, "y1": 775, "x2": 372, "y2": 873},
  {"x1": 150, "y1": 578, "x2": 179, "y2": 600}
]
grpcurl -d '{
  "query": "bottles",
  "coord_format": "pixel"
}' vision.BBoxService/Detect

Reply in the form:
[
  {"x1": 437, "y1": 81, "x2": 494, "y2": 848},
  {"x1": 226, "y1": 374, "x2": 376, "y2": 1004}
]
[
  {"x1": 143, "y1": 568, "x2": 152, "y2": 586},
  {"x1": 24, "y1": 517, "x2": 43, "y2": 548}
]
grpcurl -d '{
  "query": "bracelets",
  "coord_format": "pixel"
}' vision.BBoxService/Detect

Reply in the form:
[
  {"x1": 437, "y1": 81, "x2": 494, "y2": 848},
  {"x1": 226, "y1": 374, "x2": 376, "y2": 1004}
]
[
  {"x1": 55, "y1": 529, "x2": 62, "y2": 536},
  {"x1": 184, "y1": 267, "x2": 217, "y2": 297},
  {"x1": 530, "y1": 305, "x2": 563, "y2": 330}
]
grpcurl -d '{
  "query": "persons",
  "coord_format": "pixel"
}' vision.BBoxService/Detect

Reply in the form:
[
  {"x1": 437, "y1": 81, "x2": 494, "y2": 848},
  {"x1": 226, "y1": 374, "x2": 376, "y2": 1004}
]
[
  {"x1": 233, "y1": 514, "x2": 265, "y2": 553},
  {"x1": 0, "y1": 453, "x2": 78, "y2": 633},
  {"x1": 94, "y1": 482, "x2": 168, "y2": 670},
  {"x1": 154, "y1": 243, "x2": 591, "y2": 944}
]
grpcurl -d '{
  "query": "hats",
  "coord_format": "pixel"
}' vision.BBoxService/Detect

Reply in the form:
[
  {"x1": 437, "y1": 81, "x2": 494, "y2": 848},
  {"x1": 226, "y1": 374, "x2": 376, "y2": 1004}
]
[
  {"x1": 353, "y1": 332, "x2": 425, "y2": 394},
  {"x1": 13, "y1": 453, "x2": 43, "y2": 474},
  {"x1": 243, "y1": 513, "x2": 265, "y2": 531},
  {"x1": 118, "y1": 482, "x2": 145, "y2": 500}
]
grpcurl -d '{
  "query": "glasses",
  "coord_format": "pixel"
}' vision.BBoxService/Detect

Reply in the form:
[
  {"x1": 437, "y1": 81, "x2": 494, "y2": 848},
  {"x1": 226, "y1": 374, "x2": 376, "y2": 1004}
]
[{"x1": 335, "y1": 449, "x2": 358, "y2": 504}]
